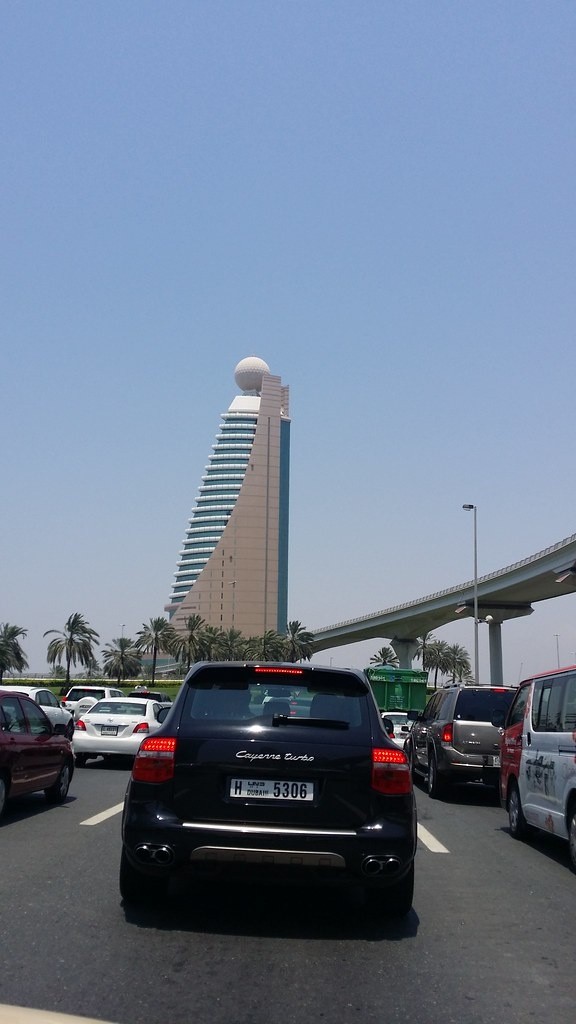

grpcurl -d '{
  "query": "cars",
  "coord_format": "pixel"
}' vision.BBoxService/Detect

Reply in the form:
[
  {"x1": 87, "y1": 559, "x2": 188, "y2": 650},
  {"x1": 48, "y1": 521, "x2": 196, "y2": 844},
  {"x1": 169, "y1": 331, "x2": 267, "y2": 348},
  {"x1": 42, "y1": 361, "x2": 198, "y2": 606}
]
[
  {"x1": 381, "y1": 712, "x2": 414, "y2": 748},
  {"x1": 1, "y1": 691, "x2": 77, "y2": 820},
  {"x1": 72, "y1": 697, "x2": 163, "y2": 768}
]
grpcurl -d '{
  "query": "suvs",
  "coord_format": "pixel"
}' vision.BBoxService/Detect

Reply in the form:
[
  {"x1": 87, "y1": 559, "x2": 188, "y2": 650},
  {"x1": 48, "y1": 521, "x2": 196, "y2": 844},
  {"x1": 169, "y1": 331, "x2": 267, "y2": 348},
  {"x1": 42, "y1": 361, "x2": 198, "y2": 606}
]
[
  {"x1": 118, "y1": 661, "x2": 418, "y2": 940},
  {"x1": 403, "y1": 685, "x2": 520, "y2": 798},
  {"x1": 128, "y1": 691, "x2": 170, "y2": 704},
  {"x1": 0, "y1": 686, "x2": 74, "y2": 741},
  {"x1": 61, "y1": 686, "x2": 126, "y2": 727}
]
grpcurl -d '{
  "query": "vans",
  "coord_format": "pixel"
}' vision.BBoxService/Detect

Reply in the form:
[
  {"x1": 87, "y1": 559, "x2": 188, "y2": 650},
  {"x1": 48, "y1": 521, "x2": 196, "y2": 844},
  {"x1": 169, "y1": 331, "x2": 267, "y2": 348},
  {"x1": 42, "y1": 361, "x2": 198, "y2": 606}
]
[{"x1": 491, "y1": 666, "x2": 575, "y2": 863}]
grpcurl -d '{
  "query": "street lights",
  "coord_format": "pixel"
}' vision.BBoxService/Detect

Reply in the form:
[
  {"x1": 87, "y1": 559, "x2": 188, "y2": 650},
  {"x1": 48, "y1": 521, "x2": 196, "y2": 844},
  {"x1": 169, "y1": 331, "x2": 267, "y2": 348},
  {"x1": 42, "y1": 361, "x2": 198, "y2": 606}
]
[
  {"x1": 122, "y1": 625, "x2": 126, "y2": 640},
  {"x1": 462, "y1": 505, "x2": 478, "y2": 684}
]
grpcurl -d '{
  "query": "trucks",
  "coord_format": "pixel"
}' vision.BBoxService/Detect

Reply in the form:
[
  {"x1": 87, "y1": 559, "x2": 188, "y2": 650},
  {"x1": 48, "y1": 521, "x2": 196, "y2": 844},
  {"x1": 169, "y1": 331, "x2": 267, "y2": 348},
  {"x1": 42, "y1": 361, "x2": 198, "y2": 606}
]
[{"x1": 364, "y1": 669, "x2": 428, "y2": 721}]
